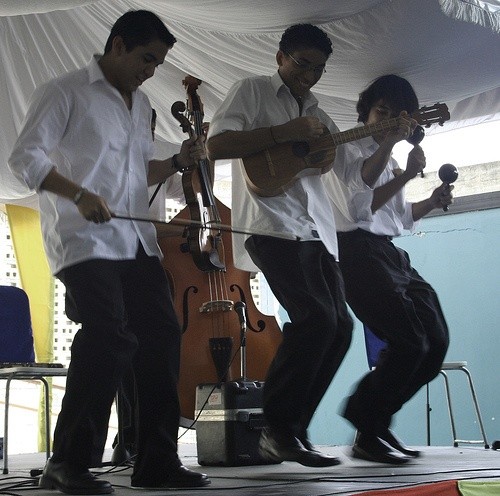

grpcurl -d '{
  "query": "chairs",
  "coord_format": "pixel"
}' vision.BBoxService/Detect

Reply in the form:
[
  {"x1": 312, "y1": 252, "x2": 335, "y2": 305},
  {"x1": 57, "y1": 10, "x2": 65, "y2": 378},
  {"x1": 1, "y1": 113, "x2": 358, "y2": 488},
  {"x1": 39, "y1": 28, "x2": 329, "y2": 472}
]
[
  {"x1": 348, "y1": 322, "x2": 489, "y2": 450},
  {"x1": 0, "y1": 286, "x2": 68, "y2": 475}
]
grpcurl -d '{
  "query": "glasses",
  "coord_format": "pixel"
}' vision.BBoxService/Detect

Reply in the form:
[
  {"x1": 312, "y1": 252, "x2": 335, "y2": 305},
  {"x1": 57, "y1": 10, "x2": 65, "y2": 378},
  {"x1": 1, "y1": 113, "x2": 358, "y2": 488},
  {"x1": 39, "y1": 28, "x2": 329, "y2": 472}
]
[{"x1": 288, "y1": 51, "x2": 327, "y2": 74}]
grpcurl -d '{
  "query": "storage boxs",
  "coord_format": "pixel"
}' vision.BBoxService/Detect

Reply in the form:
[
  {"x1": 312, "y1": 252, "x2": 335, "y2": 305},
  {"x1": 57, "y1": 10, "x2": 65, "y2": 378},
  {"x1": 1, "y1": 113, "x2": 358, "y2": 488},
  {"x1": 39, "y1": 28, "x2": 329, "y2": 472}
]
[{"x1": 195, "y1": 381, "x2": 283, "y2": 466}]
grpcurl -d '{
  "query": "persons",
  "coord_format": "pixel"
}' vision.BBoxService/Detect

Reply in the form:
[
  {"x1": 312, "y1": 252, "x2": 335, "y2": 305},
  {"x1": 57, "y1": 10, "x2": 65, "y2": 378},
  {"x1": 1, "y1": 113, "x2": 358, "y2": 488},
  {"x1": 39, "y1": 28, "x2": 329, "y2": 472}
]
[
  {"x1": 6, "y1": 11, "x2": 212, "y2": 495},
  {"x1": 206, "y1": 24, "x2": 417, "y2": 469},
  {"x1": 333, "y1": 75, "x2": 454, "y2": 465}
]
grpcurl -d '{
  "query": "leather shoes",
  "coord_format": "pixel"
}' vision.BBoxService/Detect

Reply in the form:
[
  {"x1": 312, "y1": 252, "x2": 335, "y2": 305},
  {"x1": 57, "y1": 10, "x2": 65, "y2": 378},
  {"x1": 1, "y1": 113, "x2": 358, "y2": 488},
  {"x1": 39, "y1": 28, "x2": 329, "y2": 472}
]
[
  {"x1": 130, "y1": 460, "x2": 212, "y2": 488},
  {"x1": 39, "y1": 457, "x2": 115, "y2": 495},
  {"x1": 339, "y1": 392, "x2": 421, "y2": 458},
  {"x1": 257, "y1": 429, "x2": 341, "y2": 468},
  {"x1": 351, "y1": 431, "x2": 411, "y2": 464}
]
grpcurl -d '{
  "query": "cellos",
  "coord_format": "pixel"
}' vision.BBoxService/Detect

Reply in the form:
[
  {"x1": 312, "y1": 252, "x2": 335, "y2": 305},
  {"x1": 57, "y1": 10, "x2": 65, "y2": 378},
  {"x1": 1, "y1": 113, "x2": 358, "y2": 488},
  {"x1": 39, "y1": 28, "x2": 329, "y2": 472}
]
[{"x1": 151, "y1": 75, "x2": 282, "y2": 430}]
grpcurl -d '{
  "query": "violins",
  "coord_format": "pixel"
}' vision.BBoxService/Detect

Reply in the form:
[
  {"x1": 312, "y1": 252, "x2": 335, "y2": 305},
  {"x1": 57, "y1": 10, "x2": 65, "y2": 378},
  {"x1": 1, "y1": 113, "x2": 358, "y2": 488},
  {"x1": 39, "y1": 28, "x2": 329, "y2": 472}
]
[
  {"x1": 238, "y1": 100, "x2": 451, "y2": 197},
  {"x1": 171, "y1": 101, "x2": 226, "y2": 272}
]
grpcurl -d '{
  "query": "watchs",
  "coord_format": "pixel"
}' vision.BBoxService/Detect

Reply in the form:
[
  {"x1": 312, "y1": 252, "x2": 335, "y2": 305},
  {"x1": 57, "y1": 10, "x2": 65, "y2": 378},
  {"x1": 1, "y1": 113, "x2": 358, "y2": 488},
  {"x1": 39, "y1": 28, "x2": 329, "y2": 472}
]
[{"x1": 72, "y1": 187, "x2": 89, "y2": 204}]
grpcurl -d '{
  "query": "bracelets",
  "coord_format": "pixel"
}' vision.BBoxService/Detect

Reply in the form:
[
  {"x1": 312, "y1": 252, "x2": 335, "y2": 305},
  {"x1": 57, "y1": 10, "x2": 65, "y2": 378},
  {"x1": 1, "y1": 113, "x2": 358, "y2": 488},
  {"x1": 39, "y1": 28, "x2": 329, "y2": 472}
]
[
  {"x1": 269, "y1": 127, "x2": 278, "y2": 148},
  {"x1": 171, "y1": 154, "x2": 189, "y2": 173}
]
